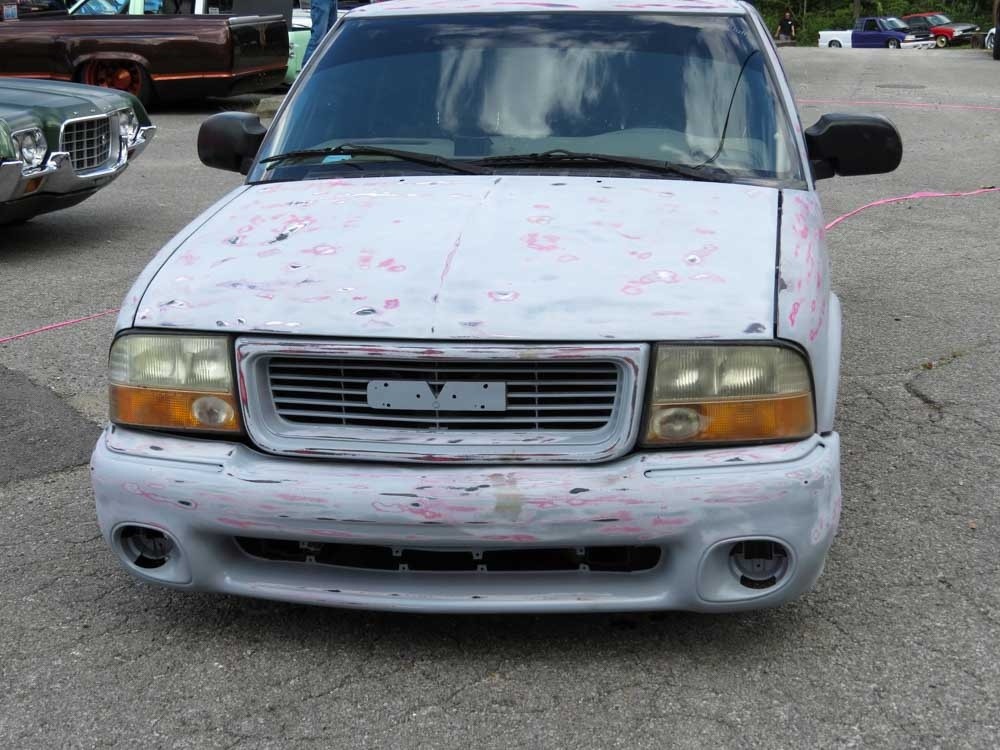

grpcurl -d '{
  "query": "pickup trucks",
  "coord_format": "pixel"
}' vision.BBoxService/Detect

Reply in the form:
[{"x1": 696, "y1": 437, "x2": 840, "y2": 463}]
[
  {"x1": 817, "y1": 16, "x2": 936, "y2": 50},
  {"x1": 0, "y1": 0, "x2": 290, "y2": 110}
]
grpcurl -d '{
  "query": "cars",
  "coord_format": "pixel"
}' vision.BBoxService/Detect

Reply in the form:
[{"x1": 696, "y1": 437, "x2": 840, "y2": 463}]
[
  {"x1": 86, "y1": 1, "x2": 904, "y2": 621},
  {"x1": 1, "y1": 77, "x2": 159, "y2": 209},
  {"x1": 901, "y1": 11, "x2": 982, "y2": 47}
]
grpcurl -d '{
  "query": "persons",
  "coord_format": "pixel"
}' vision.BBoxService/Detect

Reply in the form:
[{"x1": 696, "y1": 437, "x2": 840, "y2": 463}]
[
  {"x1": 297, "y1": 0, "x2": 339, "y2": 70},
  {"x1": 773, "y1": 11, "x2": 795, "y2": 41}
]
